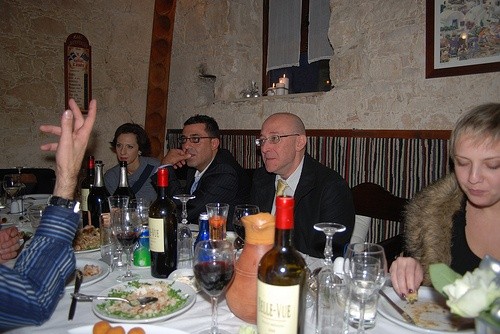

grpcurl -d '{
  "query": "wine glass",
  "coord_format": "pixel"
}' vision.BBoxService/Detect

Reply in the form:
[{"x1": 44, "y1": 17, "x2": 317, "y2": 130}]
[
  {"x1": 173, "y1": 195, "x2": 196, "y2": 269},
  {"x1": 313, "y1": 223, "x2": 387, "y2": 334},
  {"x1": 99, "y1": 196, "x2": 151, "y2": 282},
  {"x1": 193, "y1": 239, "x2": 235, "y2": 334},
  {"x1": 2, "y1": 174, "x2": 22, "y2": 203}
]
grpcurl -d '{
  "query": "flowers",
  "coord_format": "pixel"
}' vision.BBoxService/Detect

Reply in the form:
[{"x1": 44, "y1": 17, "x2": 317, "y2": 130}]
[{"x1": 429, "y1": 255, "x2": 500, "y2": 334}]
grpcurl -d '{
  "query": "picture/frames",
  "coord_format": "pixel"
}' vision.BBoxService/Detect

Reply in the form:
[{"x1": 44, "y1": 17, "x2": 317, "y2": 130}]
[{"x1": 426, "y1": 0, "x2": 500, "y2": 78}]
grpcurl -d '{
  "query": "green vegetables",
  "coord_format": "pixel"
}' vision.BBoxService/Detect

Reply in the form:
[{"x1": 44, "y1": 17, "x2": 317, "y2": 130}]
[{"x1": 97, "y1": 280, "x2": 187, "y2": 319}]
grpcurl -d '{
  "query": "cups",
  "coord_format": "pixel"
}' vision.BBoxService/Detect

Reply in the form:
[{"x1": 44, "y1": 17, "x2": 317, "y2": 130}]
[{"x1": 234, "y1": 205, "x2": 260, "y2": 243}]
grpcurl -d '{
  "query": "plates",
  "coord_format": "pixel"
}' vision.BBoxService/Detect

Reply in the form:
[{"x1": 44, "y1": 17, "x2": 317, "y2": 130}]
[
  {"x1": 63, "y1": 257, "x2": 110, "y2": 290},
  {"x1": 92, "y1": 278, "x2": 197, "y2": 324},
  {"x1": 69, "y1": 323, "x2": 188, "y2": 334},
  {"x1": 0, "y1": 213, "x2": 28, "y2": 226},
  {"x1": 74, "y1": 243, "x2": 112, "y2": 254},
  {"x1": 377, "y1": 285, "x2": 477, "y2": 334},
  {"x1": 19, "y1": 193, "x2": 52, "y2": 205},
  {"x1": 167, "y1": 269, "x2": 201, "y2": 293}
]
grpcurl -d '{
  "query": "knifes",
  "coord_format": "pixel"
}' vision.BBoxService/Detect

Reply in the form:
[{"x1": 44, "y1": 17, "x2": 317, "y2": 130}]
[
  {"x1": 379, "y1": 290, "x2": 415, "y2": 326},
  {"x1": 68, "y1": 271, "x2": 83, "y2": 320}
]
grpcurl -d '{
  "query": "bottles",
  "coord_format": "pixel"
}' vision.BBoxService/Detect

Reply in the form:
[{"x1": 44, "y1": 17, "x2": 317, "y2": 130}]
[
  {"x1": 10, "y1": 196, "x2": 24, "y2": 213},
  {"x1": 205, "y1": 203, "x2": 229, "y2": 240},
  {"x1": 80, "y1": 155, "x2": 112, "y2": 228},
  {"x1": 256, "y1": 195, "x2": 307, "y2": 334},
  {"x1": 148, "y1": 168, "x2": 177, "y2": 279},
  {"x1": 112, "y1": 160, "x2": 137, "y2": 248},
  {"x1": 193, "y1": 213, "x2": 214, "y2": 262}
]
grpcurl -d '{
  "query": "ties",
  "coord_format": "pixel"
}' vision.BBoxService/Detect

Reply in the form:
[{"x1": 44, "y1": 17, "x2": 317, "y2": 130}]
[{"x1": 276, "y1": 180, "x2": 286, "y2": 197}]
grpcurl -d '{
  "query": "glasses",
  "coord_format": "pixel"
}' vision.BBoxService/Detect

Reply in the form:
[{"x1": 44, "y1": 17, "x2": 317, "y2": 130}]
[
  {"x1": 177, "y1": 136, "x2": 218, "y2": 144},
  {"x1": 255, "y1": 134, "x2": 299, "y2": 146}
]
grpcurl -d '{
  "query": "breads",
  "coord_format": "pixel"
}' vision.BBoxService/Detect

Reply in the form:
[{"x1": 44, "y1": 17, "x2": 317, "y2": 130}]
[{"x1": 92, "y1": 321, "x2": 146, "y2": 334}]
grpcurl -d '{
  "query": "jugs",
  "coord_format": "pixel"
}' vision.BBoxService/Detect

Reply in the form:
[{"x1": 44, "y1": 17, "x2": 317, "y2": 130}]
[{"x1": 224, "y1": 212, "x2": 275, "y2": 325}]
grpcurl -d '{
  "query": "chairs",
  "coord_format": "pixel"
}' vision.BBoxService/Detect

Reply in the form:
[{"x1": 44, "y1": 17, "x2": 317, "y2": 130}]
[
  {"x1": 0, "y1": 167, "x2": 56, "y2": 196},
  {"x1": 350, "y1": 182, "x2": 411, "y2": 275}
]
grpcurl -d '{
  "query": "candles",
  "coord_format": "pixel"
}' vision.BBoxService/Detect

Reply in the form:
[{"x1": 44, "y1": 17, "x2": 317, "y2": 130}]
[{"x1": 268, "y1": 74, "x2": 289, "y2": 95}]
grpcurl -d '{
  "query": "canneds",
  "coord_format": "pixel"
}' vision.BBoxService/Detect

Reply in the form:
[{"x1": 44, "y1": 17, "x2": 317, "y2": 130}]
[{"x1": 132, "y1": 225, "x2": 152, "y2": 267}]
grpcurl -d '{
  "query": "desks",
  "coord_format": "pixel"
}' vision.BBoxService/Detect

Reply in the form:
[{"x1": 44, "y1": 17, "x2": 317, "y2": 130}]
[{"x1": 0, "y1": 195, "x2": 476, "y2": 334}]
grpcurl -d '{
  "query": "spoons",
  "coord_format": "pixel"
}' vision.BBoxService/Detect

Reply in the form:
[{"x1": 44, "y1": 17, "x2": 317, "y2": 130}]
[{"x1": 70, "y1": 292, "x2": 159, "y2": 307}]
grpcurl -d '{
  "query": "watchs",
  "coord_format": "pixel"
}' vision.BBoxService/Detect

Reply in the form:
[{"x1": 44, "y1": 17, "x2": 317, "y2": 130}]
[{"x1": 47, "y1": 194, "x2": 81, "y2": 213}]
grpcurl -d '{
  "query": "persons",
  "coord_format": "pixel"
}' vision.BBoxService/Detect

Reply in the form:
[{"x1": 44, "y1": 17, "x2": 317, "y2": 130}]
[
  {"x1": 246, "y1": 113, "x2": 355, "y2": 261},
  {"x1": 0, "y1": 98, "x2": 97, "y2": 333},
  {"x1": 150, "y1": 114, "x2": 251, "y2": 231},
  {"x1": 388, "y1": 104, "x2": 500, "y2": 299},
  {"x1": 104, "y1": 122, "x2": 161, "y2": 207}
]
